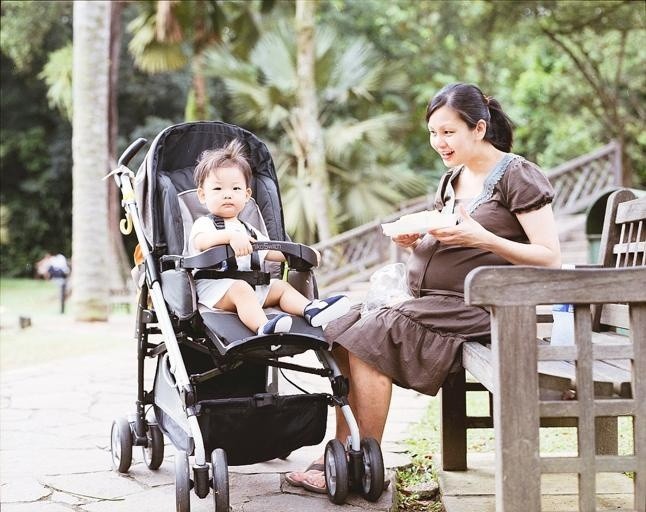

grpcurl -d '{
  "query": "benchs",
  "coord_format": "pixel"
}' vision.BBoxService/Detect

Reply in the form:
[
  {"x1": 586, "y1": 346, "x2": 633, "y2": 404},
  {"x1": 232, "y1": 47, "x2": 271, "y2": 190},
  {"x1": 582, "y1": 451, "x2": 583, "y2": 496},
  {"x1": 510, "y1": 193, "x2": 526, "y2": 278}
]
[{"x1": 440, "y1": 188, "x2": 646, "y2": 512}]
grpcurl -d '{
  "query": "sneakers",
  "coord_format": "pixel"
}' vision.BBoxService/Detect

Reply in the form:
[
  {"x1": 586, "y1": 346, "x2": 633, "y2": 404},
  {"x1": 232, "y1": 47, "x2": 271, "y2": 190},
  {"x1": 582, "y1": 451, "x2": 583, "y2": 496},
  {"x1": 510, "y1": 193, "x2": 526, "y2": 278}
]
[
  {"x1": 303, "y1": 295, "x2": 352, "y2": 328},
  {"x1": 257, "y1": 312, "x2": 293, "y2": 336}
]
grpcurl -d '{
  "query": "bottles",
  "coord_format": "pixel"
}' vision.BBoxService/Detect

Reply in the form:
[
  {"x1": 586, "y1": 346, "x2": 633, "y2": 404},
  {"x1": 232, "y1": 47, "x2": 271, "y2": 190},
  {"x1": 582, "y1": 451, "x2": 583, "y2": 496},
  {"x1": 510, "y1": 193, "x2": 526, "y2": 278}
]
[{"x1": 549, "y1": 263, "x2": 576, "y2": 348}]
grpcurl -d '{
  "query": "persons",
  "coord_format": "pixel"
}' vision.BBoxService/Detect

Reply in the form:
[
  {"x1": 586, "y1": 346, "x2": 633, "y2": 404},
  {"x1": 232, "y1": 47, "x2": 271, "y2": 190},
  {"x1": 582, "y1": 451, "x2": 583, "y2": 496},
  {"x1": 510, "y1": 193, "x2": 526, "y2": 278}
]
[
  {"x1": 285, "y1": 82, "x2": 561, "y2": 493},
  {"x1": 33, "y1": 248, "x2": 71, "y2": 282},
  {"x1": 189, "y1": 137, "x2": 350, "y2": 336}
]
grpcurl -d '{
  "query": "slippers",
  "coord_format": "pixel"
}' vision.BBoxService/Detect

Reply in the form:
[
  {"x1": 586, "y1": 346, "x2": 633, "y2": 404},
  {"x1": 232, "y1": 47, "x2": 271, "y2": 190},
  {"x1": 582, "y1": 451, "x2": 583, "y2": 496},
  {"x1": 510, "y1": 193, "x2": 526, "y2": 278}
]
[
  {"x1": 285, "y1": 458, "x2": 325, "y2": 486},
  {"x1": 301, "y1": 473, "x2": 391, "y2": 494}
]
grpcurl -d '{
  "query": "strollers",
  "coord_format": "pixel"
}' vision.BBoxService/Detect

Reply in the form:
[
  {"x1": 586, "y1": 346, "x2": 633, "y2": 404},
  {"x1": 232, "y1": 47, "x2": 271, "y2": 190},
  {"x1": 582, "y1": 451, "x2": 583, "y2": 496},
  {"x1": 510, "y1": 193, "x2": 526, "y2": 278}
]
[{"x1": 111, "y1": 121, "x2": 384, "y2": 511}]
